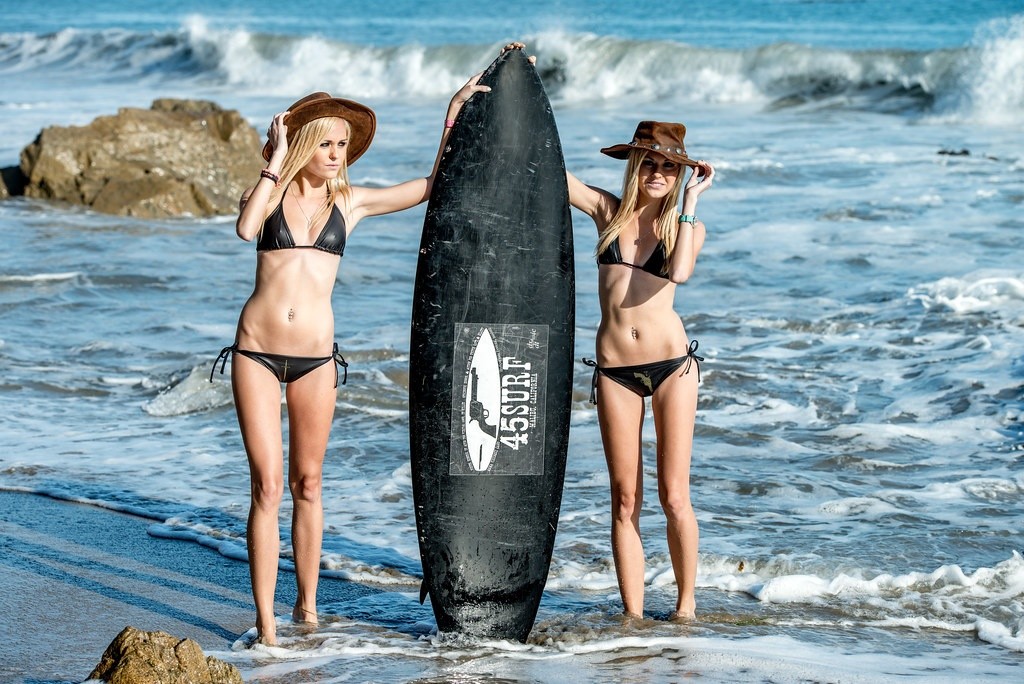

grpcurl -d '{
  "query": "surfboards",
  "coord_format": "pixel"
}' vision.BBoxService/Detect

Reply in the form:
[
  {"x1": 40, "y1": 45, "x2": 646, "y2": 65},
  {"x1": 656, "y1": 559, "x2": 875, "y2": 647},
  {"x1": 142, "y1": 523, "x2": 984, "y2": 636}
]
[{"x1": 408, "y1": 48, "x2": 577, "y2": 643}]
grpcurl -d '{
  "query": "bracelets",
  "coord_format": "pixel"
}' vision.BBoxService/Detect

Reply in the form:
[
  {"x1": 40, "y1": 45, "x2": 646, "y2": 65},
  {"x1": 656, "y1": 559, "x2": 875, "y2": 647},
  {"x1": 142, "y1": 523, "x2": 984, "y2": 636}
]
[
  {"x1": 445, "y1": 120, "x2": 456, "y2": 128},
  {"x1": 260, "y1": 169, "x2": 282, "y2": 187}
]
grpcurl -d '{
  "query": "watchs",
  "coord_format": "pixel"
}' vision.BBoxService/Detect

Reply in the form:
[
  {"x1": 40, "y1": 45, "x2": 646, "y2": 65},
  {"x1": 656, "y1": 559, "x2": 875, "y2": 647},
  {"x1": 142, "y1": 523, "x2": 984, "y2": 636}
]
[{"x1": 678, "y1": 214, "x2": 699, "y2": 228}]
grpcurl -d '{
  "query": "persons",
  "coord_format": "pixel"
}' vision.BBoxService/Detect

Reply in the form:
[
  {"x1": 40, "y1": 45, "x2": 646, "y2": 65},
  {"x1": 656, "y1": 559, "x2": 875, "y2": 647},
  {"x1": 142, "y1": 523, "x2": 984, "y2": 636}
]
[
  {"x1": 500, "y1": 41, "x2": 714, "y2": 621},
  {"x1": 209, "y1": 71, "x2": 490, "y2": 644}
]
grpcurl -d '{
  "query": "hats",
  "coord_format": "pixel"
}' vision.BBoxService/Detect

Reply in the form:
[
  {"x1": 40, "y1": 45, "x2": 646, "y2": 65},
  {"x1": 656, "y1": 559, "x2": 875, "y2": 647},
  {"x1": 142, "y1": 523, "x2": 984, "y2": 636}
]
[
  {"x1": 262, "y1": 92, "x2": 376, "y2": 166},
  {"x1": 600, "y1": 121, "x2": 706, "y2": 178}
]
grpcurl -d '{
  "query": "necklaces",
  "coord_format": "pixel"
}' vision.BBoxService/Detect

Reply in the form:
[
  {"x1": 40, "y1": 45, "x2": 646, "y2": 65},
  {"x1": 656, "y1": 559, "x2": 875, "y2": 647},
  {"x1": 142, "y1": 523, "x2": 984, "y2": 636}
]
[
  {"x1": 629, "y1": 212, "x2": 656, "y2": 244},
  {"x1": 290, "y1": 179, "x2": 328, "y2": 229}
]
